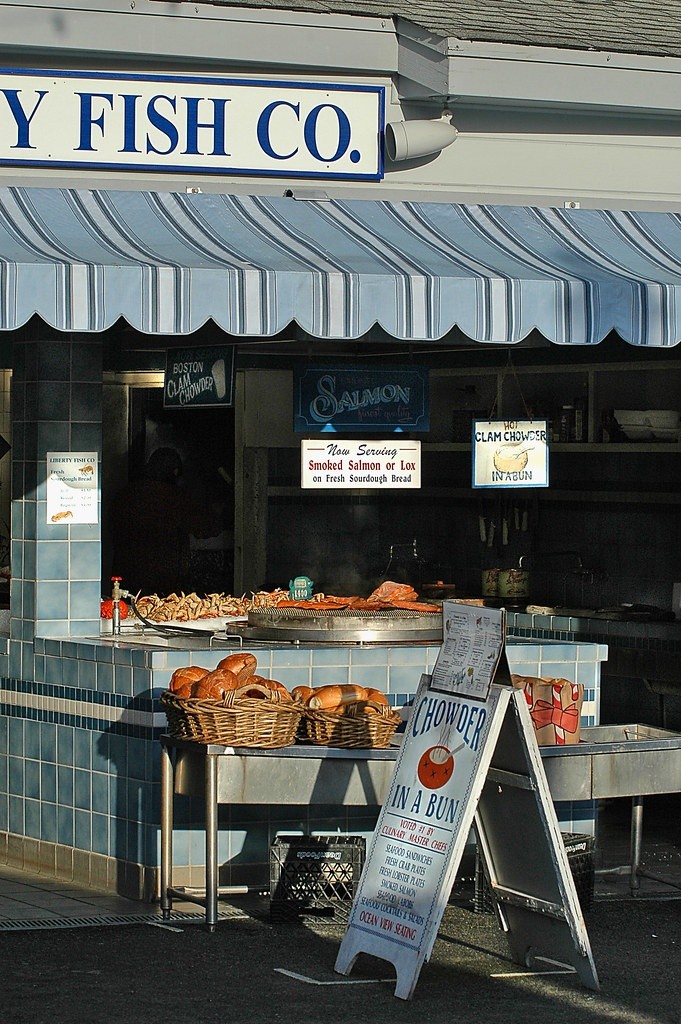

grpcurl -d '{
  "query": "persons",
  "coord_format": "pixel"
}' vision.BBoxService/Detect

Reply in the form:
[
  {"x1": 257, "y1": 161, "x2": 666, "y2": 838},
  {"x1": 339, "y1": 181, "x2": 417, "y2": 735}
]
[{"x1": 109, "y1": 447, "x2": 203, "y2": 605}]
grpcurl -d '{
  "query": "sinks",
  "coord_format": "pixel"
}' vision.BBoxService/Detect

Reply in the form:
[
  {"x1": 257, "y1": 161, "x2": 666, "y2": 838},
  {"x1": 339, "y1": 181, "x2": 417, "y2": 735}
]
[{"x1": 85, "y1": 630, "x2": 272, "y2": 650}]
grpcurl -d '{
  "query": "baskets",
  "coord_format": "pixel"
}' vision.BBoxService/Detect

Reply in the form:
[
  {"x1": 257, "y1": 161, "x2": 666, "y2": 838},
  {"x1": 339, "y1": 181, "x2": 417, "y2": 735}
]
[
  {"x1": 158, "y1": 684, "x2": 302, "y2": 749},
  {"x1": 303, "y1": 701, "x2": 403, "y2": 749}
]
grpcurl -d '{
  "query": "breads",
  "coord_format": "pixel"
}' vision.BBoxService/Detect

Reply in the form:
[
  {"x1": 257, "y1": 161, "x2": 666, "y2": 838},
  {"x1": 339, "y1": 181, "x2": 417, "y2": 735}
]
[
  {"x1": 167, "y1": 652, "x2": 294, "y2": 703},
  {"x1": 292, "y1": 684, "x2": 389, "y2": 715}
]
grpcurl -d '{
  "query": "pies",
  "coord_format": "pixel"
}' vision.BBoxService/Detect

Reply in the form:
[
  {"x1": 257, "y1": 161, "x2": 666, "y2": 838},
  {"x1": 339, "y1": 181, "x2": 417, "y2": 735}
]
[{"x1": 279, "y1": 581, "x2": 443, "y2": 612}]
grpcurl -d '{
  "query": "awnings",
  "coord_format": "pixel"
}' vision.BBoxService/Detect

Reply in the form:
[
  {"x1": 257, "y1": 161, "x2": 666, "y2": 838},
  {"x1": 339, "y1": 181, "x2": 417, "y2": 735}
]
[{"x1": 0, "y1": 186, "x2": 681, "y2": 349}]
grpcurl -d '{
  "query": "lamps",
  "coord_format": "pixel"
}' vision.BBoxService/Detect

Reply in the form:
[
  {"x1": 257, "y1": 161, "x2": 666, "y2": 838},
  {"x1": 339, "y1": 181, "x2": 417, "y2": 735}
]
[{"x1": 383, "y1": 101, "x2": 461, "y2": 162}]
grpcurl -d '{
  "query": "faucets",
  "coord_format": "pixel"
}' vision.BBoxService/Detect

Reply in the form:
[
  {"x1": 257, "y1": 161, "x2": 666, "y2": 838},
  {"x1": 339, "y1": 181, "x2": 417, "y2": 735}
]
[{"x1": 111, "y1": 577, "x2": 136, "y2": 603}]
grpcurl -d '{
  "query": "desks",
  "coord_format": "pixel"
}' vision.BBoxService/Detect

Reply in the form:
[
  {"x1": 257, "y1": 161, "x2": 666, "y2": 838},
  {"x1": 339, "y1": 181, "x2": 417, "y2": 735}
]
[{"x1": 157, "y1": 729, "x2": 403, "y2": 929}]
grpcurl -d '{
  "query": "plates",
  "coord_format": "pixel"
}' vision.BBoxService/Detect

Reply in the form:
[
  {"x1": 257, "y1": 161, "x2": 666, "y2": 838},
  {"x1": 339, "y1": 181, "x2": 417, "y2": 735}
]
[{"x1": 611, "y1": 408, "x2": 681, "y2": 441}]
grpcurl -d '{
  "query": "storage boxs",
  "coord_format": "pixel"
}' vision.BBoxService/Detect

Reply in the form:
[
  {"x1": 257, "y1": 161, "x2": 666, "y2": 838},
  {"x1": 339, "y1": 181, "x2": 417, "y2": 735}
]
[
  {"x1": 508, "y1": 674, "x2": 584, "y2": 747},
  {"x1": 473, "y1": 834, "x2": 596, "y2": 917},
  {"x1": 268, "y1": 834, "x2": 366, "y2": 925}
]
